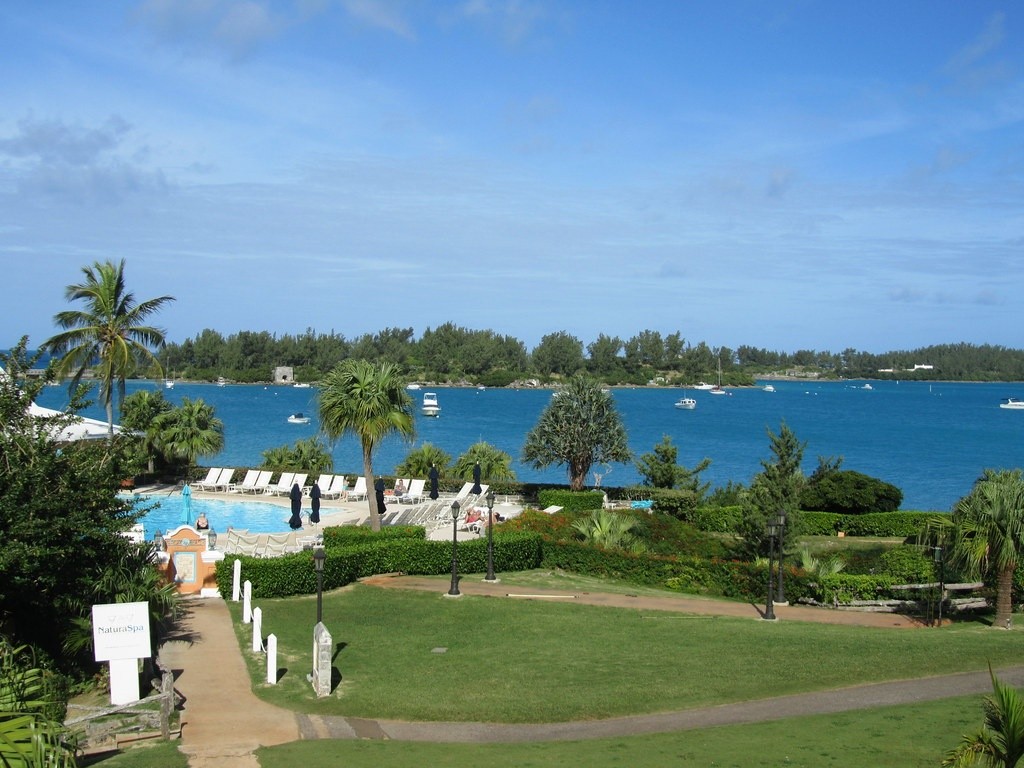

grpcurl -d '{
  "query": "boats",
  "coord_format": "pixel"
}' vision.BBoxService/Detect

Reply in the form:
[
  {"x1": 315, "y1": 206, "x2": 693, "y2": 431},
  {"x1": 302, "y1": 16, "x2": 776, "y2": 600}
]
[
  {"x1": 694, "y1": 383, "x2": 716, "y2": 390},
  {"x1": 293, "y1": 383, "x2": 310, "y2": 388},
  {"x1": 674, "y1": 398, "x2": 696, "y2": 409},
  {"x1": 288, "y1": 414, "x2": 311, "y2": 424},
  {"x1": 1000, "y1": 401, "x2": 1024, "y2": 409},
  {"x1": 166, "y1": 380, "x2": 174, "y2": 388},
  {"x1": 421, "y1": 392, "x2": 442, "y2": 416},
  {"x1": 763, "y1": 384, "x2": 774, "y2": 392}
]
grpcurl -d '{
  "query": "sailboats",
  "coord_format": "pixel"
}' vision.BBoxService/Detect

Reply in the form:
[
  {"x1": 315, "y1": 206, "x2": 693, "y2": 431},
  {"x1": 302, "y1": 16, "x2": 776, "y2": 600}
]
[{"x1": 710, "y1": 358, "x2": 726, "y2": 394}]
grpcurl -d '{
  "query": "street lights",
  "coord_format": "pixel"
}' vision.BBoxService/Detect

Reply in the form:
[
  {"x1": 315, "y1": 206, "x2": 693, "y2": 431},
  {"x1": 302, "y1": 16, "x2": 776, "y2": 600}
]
[
  {"x1": 775, "y1": 508, "x2": 788, "y2": 603},
  {"x1": 485, "y1": 492, "x2": 497, "y2": 580},
  {"x1": 763, "y1": 519, "x2": 779, "y2": 619},
  {"x1": 313, "y1": 548, "x2": 328, "y2": 623},
  {"x1": 447, "y1": 501, "x2": 460, "y2": 595}
]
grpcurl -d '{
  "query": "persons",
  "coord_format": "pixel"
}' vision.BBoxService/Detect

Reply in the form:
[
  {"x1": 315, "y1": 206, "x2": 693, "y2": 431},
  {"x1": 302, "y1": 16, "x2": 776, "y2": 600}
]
[
  {"x1": 393, "y1": 479, "x2": 408, "y2": 496},
  {"x1": 338, "y1": 474, "x2": 350, "y2": 503},
  {"x1": 194, "y1": 512, "x2": 209, "y2": 531},
  {"x1": 463, "y1": 507, "x2": 506, "y2": 527}
]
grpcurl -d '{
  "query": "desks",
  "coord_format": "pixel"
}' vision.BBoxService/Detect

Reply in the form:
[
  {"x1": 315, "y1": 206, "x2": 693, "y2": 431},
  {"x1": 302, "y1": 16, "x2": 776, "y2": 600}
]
[
  {"x1": 263, "y1": 485, "x2": 277, "y2": 494},
  {"x1": 221, "y1": 483, "x2": 236, "y2": 492},
  {"x1": 302, "y1": 486, "x2": 314, "y2": 496}
]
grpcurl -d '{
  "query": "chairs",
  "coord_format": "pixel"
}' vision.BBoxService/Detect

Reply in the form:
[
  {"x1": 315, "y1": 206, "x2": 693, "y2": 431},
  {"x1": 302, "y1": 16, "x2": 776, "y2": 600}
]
[
  {"x1": 317, "y1": 474, "x2": 333, "y2": 497},
  {"x1": 278, "y1": 474, "x2": 309, "y2": 497},
  {"x1": 203, "y1": 469, "x2": 236, "y2": 492},
  {"x1": 325, "y1": 475, "x2": 344, "y2": 499},
  {"x1": 296, "y1": 495, "x2": 478, "y2": 547},
  {"x1": 474, "y1": 484, "x2": 489, "y2": 503},
  {"x1": 403, "y1": 479, "x2": 426, "y2": 504},
  {"x1": 543, "y1": 504, "x2": 564, "y2": 515},
  {"x1": 347, "y1": 476, "x2": 368, "y2": 502},
  {"x1": 234, "y1": 470, "x2": 261, "y2": 493},
  {"x1": 457, "y1": 482, "x2": 475, "y2": 498},
  {"x1": 384, "y1": 479, "x2": 410, "y2": 503},
  {"x1": 591, "y1": 489, "x2": 616, "y2": 509},
  {"x1": 273, "y1": 473, "x2": 295, "y2": 497},
  {"x1": 189, "y1": 468, "x2": 222, "y2": 491},
  {"x1": 226, "y1": 529, "x2": 292, "y2": 560},
  {"x1": 242, "y1": 471, "x2": 273, "y2": 496}
]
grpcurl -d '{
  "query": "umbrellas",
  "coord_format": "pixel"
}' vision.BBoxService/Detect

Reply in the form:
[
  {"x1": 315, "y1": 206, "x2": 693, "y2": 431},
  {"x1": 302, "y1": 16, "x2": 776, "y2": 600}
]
[
  {"x1": 374, "y1": 475, "x2": 387, "y2": 516},
  {"x1": 288, "y1": 482, "x2": 303, "y2": 531},
  {"x1": 180, "y1": 482, "x2": 194, "y2": 526},
  {"x1": 309, "y1": 480, "x2": 321, "y2": 526},
  {"x1": 469, "y1": 461, "x2": 482, "y2": 499},
  {"x1": 427, "y1": 463, "x2": 440, "y2": 499}
]
